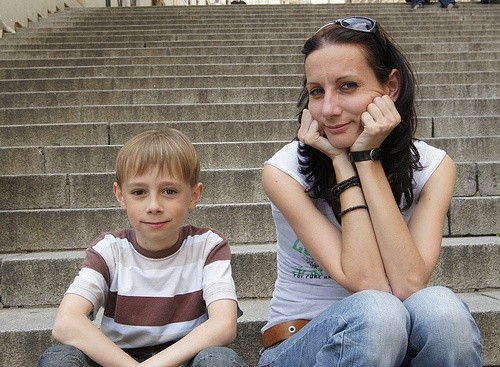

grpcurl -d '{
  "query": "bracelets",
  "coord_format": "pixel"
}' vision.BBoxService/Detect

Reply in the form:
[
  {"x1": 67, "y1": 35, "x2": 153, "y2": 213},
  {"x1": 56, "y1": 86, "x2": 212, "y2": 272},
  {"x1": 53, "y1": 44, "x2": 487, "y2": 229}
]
[
  {"x1": 331, "y1": 176, "x2": 361, "y2": 199},
  {"x1": 350, "y1": 148, "x2": 382, "y2": 163},
  {"x1": 338, "y1": 205, "x2": 368, "y2": 217}
]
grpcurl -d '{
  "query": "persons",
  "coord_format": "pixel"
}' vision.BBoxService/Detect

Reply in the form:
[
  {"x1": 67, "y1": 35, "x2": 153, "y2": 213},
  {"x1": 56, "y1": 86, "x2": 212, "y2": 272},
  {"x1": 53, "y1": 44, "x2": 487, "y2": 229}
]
[
  {"x1": 406, "y1": 0, "x2": 459, "y2": 9},
  {"x1": 38, "y1": 127, "x2": 250, "y2": 366},
  {"x1": 257, "y1": 16, "x2": 485, "y2": 367}
]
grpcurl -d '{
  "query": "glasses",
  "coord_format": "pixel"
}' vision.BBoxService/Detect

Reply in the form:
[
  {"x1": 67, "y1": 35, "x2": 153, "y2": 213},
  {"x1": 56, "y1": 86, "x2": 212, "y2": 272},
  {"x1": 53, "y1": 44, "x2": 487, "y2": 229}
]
[{"x1": 315, "y1": 16, "x2": 387, "y2": 49}]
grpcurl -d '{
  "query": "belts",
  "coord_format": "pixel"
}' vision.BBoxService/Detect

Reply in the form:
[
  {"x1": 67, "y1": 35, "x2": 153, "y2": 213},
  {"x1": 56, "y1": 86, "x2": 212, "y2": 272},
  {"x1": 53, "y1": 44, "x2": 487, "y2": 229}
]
[{"x1": 262, "y1": 320, "x2": 311, "y2": 348}]
